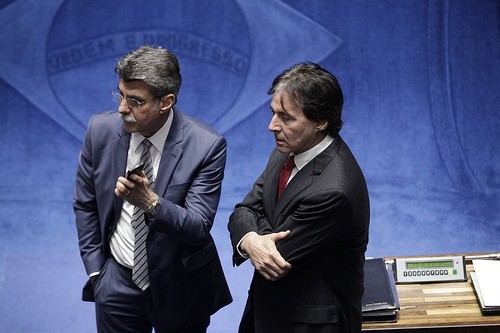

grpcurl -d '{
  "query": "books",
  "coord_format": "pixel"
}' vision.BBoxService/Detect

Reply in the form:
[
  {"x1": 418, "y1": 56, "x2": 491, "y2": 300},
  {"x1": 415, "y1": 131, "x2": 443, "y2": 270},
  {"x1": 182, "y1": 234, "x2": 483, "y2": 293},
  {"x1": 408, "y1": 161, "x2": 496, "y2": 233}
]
[
  {"x1": 361, "y1": 257, "x2": 401, "y2": 324},
  {"x1": 469, "y1": 258, "x2": 500, "y2": 315}
]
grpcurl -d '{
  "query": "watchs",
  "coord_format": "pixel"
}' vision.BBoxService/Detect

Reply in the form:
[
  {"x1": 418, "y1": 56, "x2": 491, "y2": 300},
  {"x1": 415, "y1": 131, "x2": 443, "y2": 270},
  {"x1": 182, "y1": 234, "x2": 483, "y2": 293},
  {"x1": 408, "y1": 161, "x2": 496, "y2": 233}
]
[{"x1": 147, "y1": 199, "x2": 160, "y2": 218}]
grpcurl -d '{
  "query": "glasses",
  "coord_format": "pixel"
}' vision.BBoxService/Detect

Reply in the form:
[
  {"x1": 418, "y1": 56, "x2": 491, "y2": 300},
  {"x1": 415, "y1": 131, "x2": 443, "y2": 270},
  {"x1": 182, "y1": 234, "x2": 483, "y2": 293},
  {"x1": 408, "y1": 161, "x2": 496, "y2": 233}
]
[{"x1": 112, "y1": 88, "x2": 161, "y2": 109}]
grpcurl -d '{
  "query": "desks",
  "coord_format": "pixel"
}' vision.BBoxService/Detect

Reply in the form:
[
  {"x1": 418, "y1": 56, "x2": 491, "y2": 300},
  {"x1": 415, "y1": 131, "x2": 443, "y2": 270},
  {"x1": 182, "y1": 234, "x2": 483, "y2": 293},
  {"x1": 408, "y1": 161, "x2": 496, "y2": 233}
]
[{"x1": 361, "y1": 251, "x2": 500, "y2": 333}]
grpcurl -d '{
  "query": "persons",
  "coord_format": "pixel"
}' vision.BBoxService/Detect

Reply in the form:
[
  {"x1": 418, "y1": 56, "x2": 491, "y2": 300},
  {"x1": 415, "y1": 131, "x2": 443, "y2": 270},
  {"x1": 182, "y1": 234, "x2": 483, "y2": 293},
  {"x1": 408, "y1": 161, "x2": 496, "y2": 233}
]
[
  {"x1": 72, "y1": 45, "x2": 234, "y2": 333},
  {"x1": 227, "y1": 60, "x2": 371, "y2": 333}
]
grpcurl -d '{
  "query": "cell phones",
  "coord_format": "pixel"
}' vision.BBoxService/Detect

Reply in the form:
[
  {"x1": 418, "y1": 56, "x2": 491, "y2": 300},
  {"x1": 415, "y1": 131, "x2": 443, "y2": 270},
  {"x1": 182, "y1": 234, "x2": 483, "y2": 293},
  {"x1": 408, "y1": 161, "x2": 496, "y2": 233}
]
[{"x1": 125, "y1": 162, "x2": 145, "y2": 189}]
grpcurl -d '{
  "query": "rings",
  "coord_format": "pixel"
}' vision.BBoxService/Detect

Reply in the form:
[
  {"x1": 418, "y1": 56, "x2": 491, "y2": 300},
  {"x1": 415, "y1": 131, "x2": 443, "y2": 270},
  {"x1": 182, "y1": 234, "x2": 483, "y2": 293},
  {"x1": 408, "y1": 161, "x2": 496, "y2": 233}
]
[{"x1": 124, "y1": 189, "x2": 131, "y2": 196}]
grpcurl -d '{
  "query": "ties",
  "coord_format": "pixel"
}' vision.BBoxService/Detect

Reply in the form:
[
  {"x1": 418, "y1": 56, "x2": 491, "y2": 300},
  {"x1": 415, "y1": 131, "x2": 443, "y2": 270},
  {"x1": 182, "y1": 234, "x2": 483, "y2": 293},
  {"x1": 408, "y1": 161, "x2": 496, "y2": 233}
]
[
  {"x1": 130, "y1": 139, "x2": 154, "y2": 292},
  {"x1": 278, "y1": 156, "x2": 296, "y2": 202}
]
